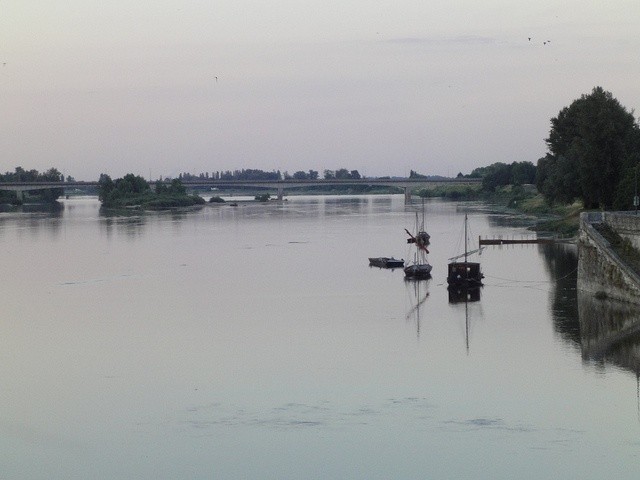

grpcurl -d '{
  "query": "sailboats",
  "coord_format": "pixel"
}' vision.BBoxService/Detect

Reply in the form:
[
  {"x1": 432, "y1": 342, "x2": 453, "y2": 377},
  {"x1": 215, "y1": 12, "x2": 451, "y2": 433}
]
[
  {"x1": 446, "y1": 212, "x2": 483, "y2": 291},
  {"x1": 404, "y1": 210, "x2": 432, "y2": 278},
  {"x1": 404, "y1": 196, "x2": 430, "y2": 243}
]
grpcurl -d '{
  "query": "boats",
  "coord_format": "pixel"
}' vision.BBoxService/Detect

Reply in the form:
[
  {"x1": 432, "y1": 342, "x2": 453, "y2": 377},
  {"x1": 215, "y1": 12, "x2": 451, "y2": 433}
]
[{"x1": 368, "y1": 256, "x2": 405, "y2": 269}]
